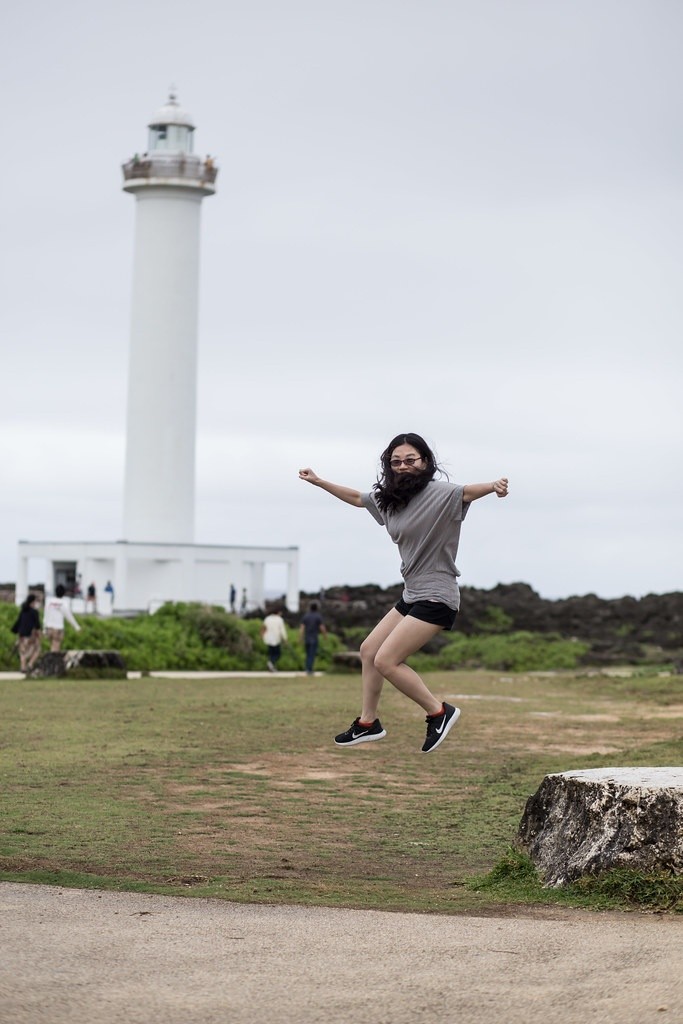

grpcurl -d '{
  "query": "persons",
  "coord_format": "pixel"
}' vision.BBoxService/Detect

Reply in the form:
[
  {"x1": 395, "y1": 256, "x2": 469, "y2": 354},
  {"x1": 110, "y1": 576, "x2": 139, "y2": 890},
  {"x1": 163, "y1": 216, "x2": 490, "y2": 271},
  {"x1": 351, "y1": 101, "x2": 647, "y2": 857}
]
[
  {"x1": 229, "y1": 585, "x2": 328, "y2": 674},
  {"x1": 298, "y1": 432, "x2": 509, "y2": 753},
  {"x1": 10, "y1": 583, "x2": 82, "y2": 672}
]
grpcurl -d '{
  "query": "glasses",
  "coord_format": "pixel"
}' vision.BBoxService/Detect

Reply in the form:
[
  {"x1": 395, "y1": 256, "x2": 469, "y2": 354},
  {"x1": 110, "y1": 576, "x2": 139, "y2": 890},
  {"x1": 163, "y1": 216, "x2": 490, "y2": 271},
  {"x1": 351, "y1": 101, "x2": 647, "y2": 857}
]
[{"x1": 390, "y1": 456, "x2": 423, "y2": 467}]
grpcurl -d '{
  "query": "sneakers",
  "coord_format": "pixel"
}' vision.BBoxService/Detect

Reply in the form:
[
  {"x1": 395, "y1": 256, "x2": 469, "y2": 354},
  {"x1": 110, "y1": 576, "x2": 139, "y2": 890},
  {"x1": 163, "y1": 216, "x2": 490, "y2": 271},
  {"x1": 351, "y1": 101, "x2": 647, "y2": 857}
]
[
  {"x1": 421, "y1": 702, "x2": 462, "y2": 754},
  {"x1": 334, "y1": 717, "x2": 386, "y2": 746}
]
